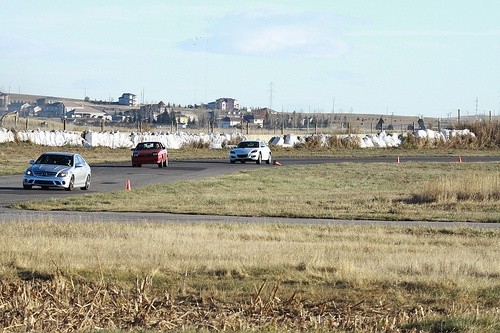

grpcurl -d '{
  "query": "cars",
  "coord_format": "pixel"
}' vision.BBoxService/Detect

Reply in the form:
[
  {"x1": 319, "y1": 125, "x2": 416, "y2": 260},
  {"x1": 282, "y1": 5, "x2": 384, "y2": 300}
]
[
  {"x1": 131, "y1": 141, "x2": 169, "y2": 168},
  {"x1": 23, "y1": 152, "x2": 91, "y2": 191},
  {"x1": 229, "y1": 140, "x2": 271, "y2": 164}
]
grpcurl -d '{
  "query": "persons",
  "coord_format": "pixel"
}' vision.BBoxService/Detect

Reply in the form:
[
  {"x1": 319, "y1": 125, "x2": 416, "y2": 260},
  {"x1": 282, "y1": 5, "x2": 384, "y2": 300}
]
[
  {"x1": 154, "y1": 144, "x2": 159, "y2": 149},
  {"x1": 64, "y1": 159, "x2": 72, "y2": 167}
]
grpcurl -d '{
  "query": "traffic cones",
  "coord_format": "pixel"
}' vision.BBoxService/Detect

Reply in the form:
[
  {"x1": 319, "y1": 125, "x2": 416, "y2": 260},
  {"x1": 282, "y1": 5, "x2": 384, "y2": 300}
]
[
  {"x1": 459, "y1": 157, "x2": 461, "y2": 162},
  {"x1": 397, "y1": 156, "x2": 400, "y2": 163},
  {"x1": 126, "y1": 178, "x2": 131, "y2": 190},
  {"x1": 458, "y1": 155, "x2": 460, "y2": 160},
  {"x1": 272, "y1": 160, "x2": 281, "y2": 166}
]
